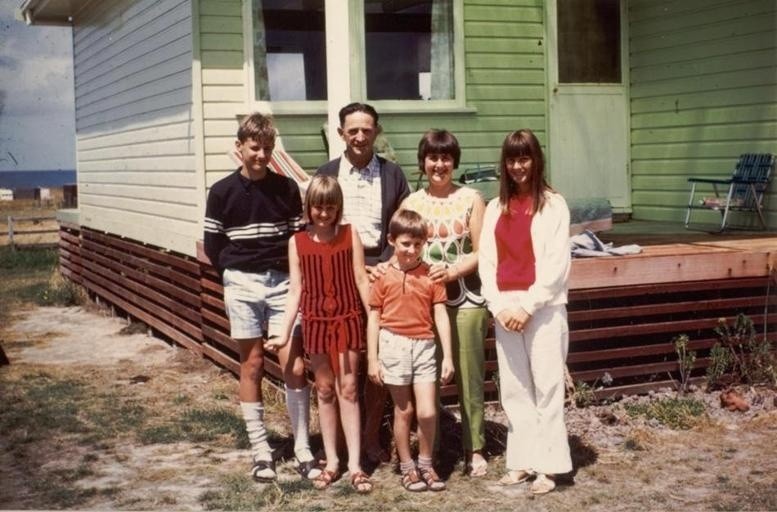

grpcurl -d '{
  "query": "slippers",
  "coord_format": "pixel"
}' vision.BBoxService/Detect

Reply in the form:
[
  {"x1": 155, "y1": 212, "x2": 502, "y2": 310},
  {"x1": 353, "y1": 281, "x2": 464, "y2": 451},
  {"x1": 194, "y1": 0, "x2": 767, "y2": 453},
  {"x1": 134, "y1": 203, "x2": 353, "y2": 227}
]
[
  {"x1": 466, "y1": 456, "x2": 489, "y2": 478},
  {"x1": 311, "y1": 468, "x2": 341, "y2": 490},
  {"x1": 348, "y1": 470, "x2": 374, "y2": 494}
]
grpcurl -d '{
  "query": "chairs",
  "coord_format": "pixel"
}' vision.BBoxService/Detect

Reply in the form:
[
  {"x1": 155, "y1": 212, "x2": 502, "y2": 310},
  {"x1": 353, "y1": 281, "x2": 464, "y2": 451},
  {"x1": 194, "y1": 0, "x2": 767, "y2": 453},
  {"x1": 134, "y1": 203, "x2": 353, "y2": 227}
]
[{"x1": 683, "y1": 153, "x2": 777, "y2": 238}]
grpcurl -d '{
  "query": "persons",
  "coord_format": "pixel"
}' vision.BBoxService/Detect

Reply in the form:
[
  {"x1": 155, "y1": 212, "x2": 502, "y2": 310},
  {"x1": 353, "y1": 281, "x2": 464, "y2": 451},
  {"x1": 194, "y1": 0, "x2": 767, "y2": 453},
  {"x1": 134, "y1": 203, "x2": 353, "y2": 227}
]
[
  {"x1": 366, "y1": 209, "x2": 455, "y2": 492},
  {"x1": 368, "y1": 128, "x2": 487, "y2": 477},
  {"x1": 314, "y1": 102, "x2": 410, "y2": 465},
  {"x1": 205, "y1": 113, "x2": 324, "y2": 482},
  {"x1": 477, "y1": 128, "x2": 573, "y2": 492},
  {"x1": 262, "y1": 174, "x2": 371, "y2": 493}
]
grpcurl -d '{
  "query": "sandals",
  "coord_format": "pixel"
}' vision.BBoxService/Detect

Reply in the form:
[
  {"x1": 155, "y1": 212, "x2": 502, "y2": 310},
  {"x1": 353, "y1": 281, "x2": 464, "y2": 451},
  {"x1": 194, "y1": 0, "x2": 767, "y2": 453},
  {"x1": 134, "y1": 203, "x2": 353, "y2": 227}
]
[
  {"x1": 248, "y1": 458, "x2": 278, "y2": 482},
  {"x1": 499, "y1": 468, "x2": 529, "y2": 485},
  {"x1": 419, "y1": 466, "x2": 446, "y2": 492},
  {"x1": 400, "y1": 470, "x2": 427, "y2": 492},
  {"x1": 530, "y1": 474, "x2": 557, "y2": 495},
  {"x1": 291, "y1": 454, "x2": 323, "y2": 481},
  {"x1": 361, "y1": 454, "x2": 382, "y2": 476}
]
